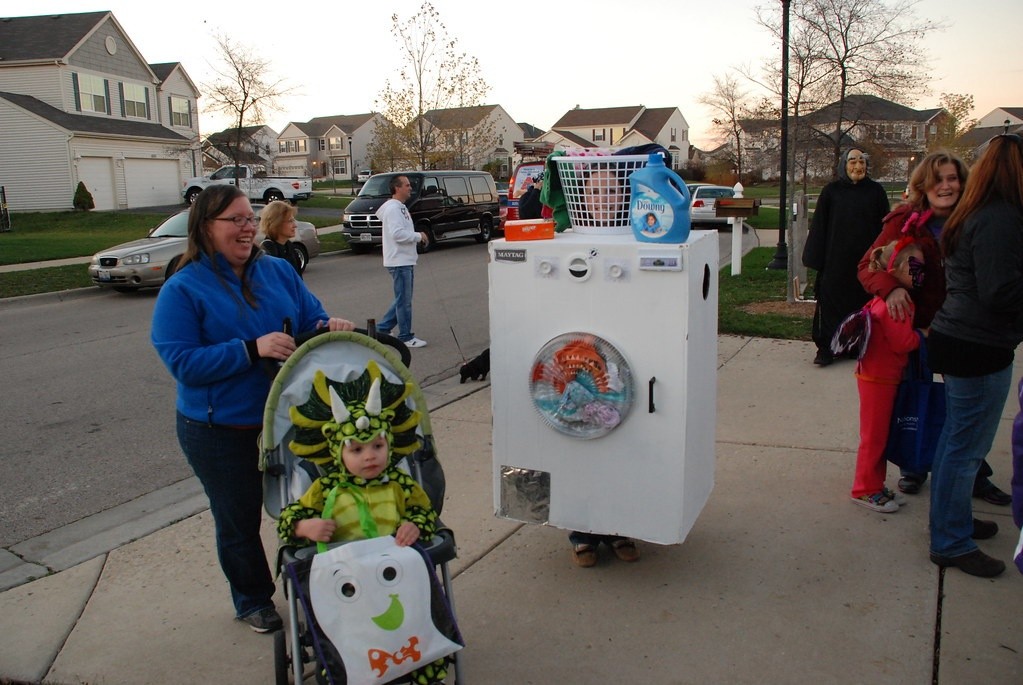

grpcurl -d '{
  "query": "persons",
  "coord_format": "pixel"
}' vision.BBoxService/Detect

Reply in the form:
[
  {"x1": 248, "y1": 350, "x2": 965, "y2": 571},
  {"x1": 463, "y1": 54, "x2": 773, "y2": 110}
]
[
  {"x1": 858, "y1": 149, "x2": 1014, "y2": 505},
  {"x1": 852, "y1": 207, "x2": 934, "y2": 513},
  {"x1": 372, "y1": 175, "x2": 430, "y2": 348},
  {"x1": 567, "y1": 162, "x2": 643, "y2": 569},
  {"x1": 149, "y1": 185, "x2": 356, "y2": 633},
  {"x1": 802, "y1": 146, "x2": 891, "y2": 366},
  {"x1": 272, "y1": 360, "x2": 464, "y2": 685},
  {"x1": 257, "y1": 200, "x2": 304, "y2": 282},
  {"x1": 927, "y1": 135, "x2": 1023, "y2": 578}
]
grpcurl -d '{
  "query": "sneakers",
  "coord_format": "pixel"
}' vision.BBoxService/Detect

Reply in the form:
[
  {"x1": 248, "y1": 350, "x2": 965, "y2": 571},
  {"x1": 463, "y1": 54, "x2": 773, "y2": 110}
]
[
  {"x1": 970, "y1": 517, "x2": 998, "y2": 540},
  {"x1": 930, "y1": 545, "x2": 1006, "y2": 577},
  {"x1": 235, "y1": 607, "x2": 283, "y2": 635},
  {"x1": 403, "y1": 337, "x2": 427, "y2": 348},
  {"x1": 851, "y1": 488, "x2": 899, "y2": 512},
  {"x1": 881, "y1": 484, "x2": 907, "y2": 506},
  {"x1": 813, "y1": 346, "x2": 833, "y2": 364}
]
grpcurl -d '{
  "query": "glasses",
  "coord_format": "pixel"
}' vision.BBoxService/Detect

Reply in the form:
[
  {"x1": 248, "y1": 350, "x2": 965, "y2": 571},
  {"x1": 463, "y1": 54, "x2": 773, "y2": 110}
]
[{"x1": 204, "y1": 216, "x2": 261, "y2": 227}]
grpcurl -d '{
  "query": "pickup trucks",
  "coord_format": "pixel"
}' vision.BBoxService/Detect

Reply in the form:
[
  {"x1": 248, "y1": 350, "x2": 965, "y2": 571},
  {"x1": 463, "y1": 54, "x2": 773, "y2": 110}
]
[{"x1": 181, "y1": 163, "x2": 312, "y2": 207}]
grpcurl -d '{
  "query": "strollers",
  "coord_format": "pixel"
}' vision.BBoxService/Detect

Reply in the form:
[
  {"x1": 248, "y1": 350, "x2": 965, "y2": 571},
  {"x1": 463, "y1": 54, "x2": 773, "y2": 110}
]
[{"x1": 258, "y1": 319, "x2": 466, "y2": 685}]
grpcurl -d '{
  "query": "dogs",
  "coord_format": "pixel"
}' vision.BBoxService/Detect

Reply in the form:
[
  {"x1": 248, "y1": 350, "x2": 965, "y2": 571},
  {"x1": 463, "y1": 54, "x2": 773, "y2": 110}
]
[{"x1": 460, "y1": 348, "x2": 490, "y2": 383}]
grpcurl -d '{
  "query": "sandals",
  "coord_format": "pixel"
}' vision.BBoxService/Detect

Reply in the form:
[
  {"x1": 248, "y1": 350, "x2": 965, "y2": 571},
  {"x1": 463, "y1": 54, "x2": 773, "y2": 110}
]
[
  {"x1": 971, "y1": 476, "x2": 1012, "y2": 506},
  {"x1": 897, "y1": 472, "x2": 929, "y2": 494}
]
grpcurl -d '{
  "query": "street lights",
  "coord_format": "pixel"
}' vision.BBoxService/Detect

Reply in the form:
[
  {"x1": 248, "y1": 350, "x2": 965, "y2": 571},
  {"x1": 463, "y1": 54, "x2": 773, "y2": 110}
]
[
  {"x1": 1004, "y1": 119, "x2": 1010, "y2": 134},
  {"x1": 348, "y1": 137, "x2": 356, "y2": 196}
]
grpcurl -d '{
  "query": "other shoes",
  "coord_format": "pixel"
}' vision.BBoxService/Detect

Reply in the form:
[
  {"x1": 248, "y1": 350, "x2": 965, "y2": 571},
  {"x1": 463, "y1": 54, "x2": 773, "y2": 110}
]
[
  {"x1": 609, "y1": 539, "x2": 640, "y2": 561},
  {"x1": 573, "y1": 544, "x2": 598, "y2": 567}
]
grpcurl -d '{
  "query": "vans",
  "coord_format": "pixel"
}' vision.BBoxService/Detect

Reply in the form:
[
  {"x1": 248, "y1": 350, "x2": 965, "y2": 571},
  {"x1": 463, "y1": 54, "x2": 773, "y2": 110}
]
[
  {"x1": 343, "y1": 171, "x2": 501, "y2": 253},
  {"x1": 507, "y1": 162, "x2": 553, "y2": 221}
]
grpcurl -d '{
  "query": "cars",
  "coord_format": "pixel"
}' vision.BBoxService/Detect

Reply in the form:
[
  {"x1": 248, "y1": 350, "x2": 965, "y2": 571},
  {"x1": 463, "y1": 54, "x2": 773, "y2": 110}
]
[
  {"x1": 357, "y1": 170, "x2": 383, "y2": 184},
  {"x1": 88, "y1": 204, "x2": 323, "y2": 294},
  {"x1": 494, "y1": 189, "x2": 509, "y2": 235},
  {"x1": 688, "y1": 186, "x2": 746, "y2": 231}
]
